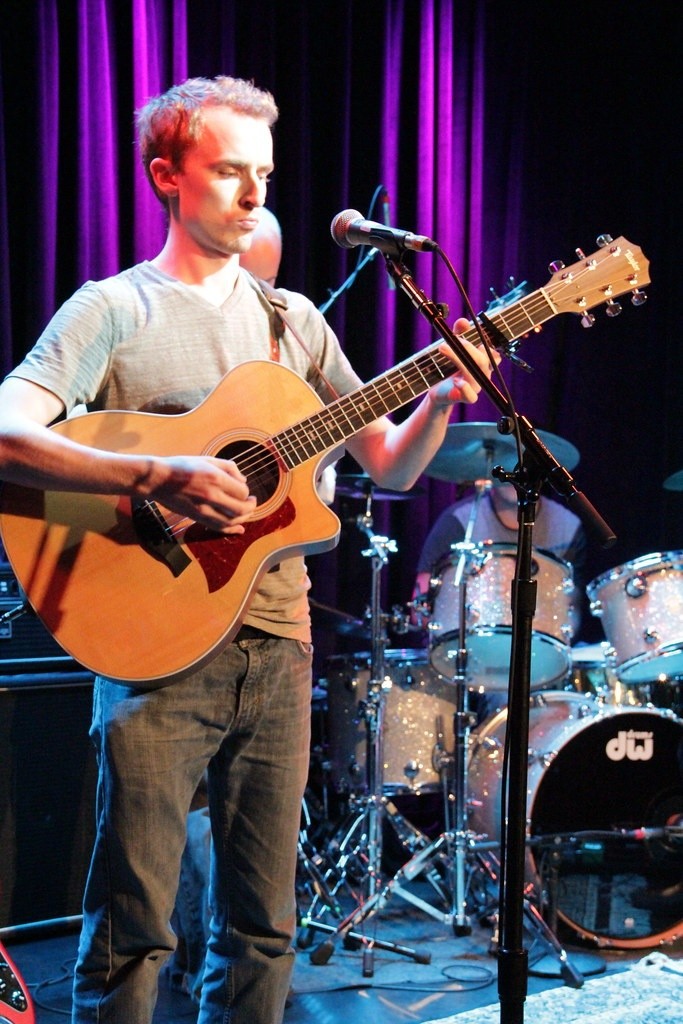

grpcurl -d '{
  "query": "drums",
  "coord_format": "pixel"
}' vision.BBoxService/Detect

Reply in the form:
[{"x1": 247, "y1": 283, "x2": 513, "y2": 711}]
[
  {"x1": 555, "y1": 642, "x2": 683, "y2": 717},
  {"x1": 586, "y1": 547, "x2": 683, "y2": 684},
  {"x1": 426, "y1": 541, "x2": 574, "y2": 690},
  {"x1": 328, "y1": 647, "x2": 469, "y2": 797},
  {"x1": 450, "y1": 688, "x2": 683, "y2": 951}
]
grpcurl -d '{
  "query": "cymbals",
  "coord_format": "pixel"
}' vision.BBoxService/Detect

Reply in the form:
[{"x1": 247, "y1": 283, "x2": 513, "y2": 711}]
[
  {"x1": 422, "y1": 422, "x2": 581, "y2": 488},
  {"x1": 335, "y1": 473, "x2": 423, "y2": 501}
]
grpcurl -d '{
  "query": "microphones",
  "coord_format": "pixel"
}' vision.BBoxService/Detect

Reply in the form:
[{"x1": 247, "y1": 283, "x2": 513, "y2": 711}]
[{"x1": 329, "y1": 209, "x2": 439, "y2": 254}]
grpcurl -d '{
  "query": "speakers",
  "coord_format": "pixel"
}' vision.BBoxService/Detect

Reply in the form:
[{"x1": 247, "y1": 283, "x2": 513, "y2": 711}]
[{"x1": 0, "y1": 672, "x2": 110, "y2": 940}]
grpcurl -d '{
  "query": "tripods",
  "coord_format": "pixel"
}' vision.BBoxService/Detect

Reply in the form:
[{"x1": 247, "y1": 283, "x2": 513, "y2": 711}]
[{"x1": 290, "y1": 448, "x2": 584, "y2": 991}]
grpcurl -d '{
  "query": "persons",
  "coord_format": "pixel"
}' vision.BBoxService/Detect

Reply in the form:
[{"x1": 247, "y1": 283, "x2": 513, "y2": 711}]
[
  {"x1": 0, "y1": 73, "x2": 503, "y2": 1024},
  {"x1": 411, "y1": 476, "x2": 592, "y2": 928}
]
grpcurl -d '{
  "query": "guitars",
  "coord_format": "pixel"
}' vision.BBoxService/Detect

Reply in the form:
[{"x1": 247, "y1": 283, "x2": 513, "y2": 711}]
[{"x1": 1, "y1": 232, "x2": 651, "y2": 691}]
